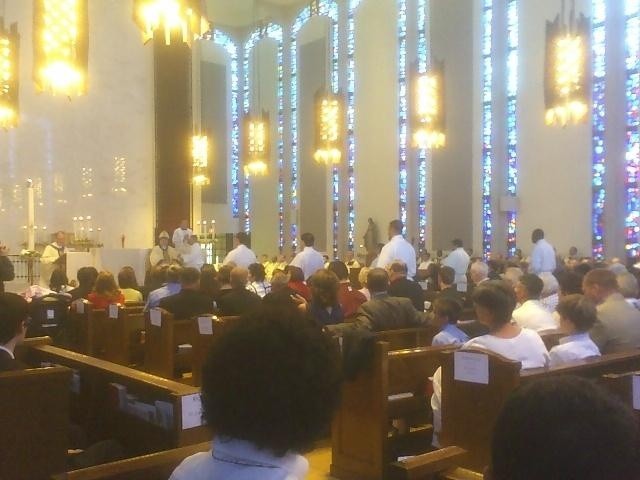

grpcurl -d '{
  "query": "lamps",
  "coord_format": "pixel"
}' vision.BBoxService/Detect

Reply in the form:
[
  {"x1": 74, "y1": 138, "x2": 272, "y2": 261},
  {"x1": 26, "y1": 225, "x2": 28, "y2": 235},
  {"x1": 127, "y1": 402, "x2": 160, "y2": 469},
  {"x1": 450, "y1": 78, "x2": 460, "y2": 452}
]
[
  {"x1": 0, "y1": 0, "x2": 211, "y2": 131},
  {"x1": 313, "y1": 90, "x2": 345, "y2": 167},
  {"x1": 407, "y1": 69, "x2": 448, "y2": 153},
  {"x1": 542, "y1": 2, "x2": 591, "y2": 131},
  {"x1": 189, "y1": 134, "x2": 210, "y2": 188},
  {"x1": 243, "y1": 116, "x2": 270, "y2": 178}
]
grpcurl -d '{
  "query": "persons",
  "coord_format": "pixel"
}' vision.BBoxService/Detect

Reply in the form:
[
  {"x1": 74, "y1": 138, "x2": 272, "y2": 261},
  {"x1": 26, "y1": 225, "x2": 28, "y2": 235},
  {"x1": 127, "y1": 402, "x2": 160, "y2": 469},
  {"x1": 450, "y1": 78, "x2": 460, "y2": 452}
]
[
  {"x1": 167, "y1": 303, "x2": 343, "y2": 480},
  {"x1": 578, "y1": 254, "x2": 640, "y2": 310},
  {"x1": 483, "y1": 374, "x2": 639, "y2": 480},
  {"x1": 527, "y1": 229, "x2": 559, "y2": 298},
  {"x1": 1, "y1": 235, "x2": 578, "y2": 450},
  {"x1": 363, "y1": 218, "x2": 376, "y2": 256},
  {"x1": 288, "y1": 233, "x2": 325, "y2": 284},
  {"x1": 376, "y1": 220, "x2": 416, "y2": 281},
  {"x1": 150, "y1": 230, "x2": 174, "y2": 267},
  {"x1": 223, "y1": 232, "x2": 256, "y2": 271},
  {"x1": 548, "y1": 293, "x2": 602, "y2": 366},
  {"x1": 171, "y1": 218, "x2": 193, "y2": 245},
  {"x1": 581, "y1": 267, "x2": 640, "y2": 353},
  {"x1": 39, "y1": 231, "x2": 68, "y2": 264}
]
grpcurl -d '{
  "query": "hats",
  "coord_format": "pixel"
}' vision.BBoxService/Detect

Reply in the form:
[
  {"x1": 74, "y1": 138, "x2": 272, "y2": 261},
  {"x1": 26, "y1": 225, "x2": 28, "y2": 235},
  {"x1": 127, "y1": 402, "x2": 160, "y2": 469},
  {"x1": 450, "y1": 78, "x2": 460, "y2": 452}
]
[{"x1": 158, "y1": 230, "x2": 169, "y2": 239}]
